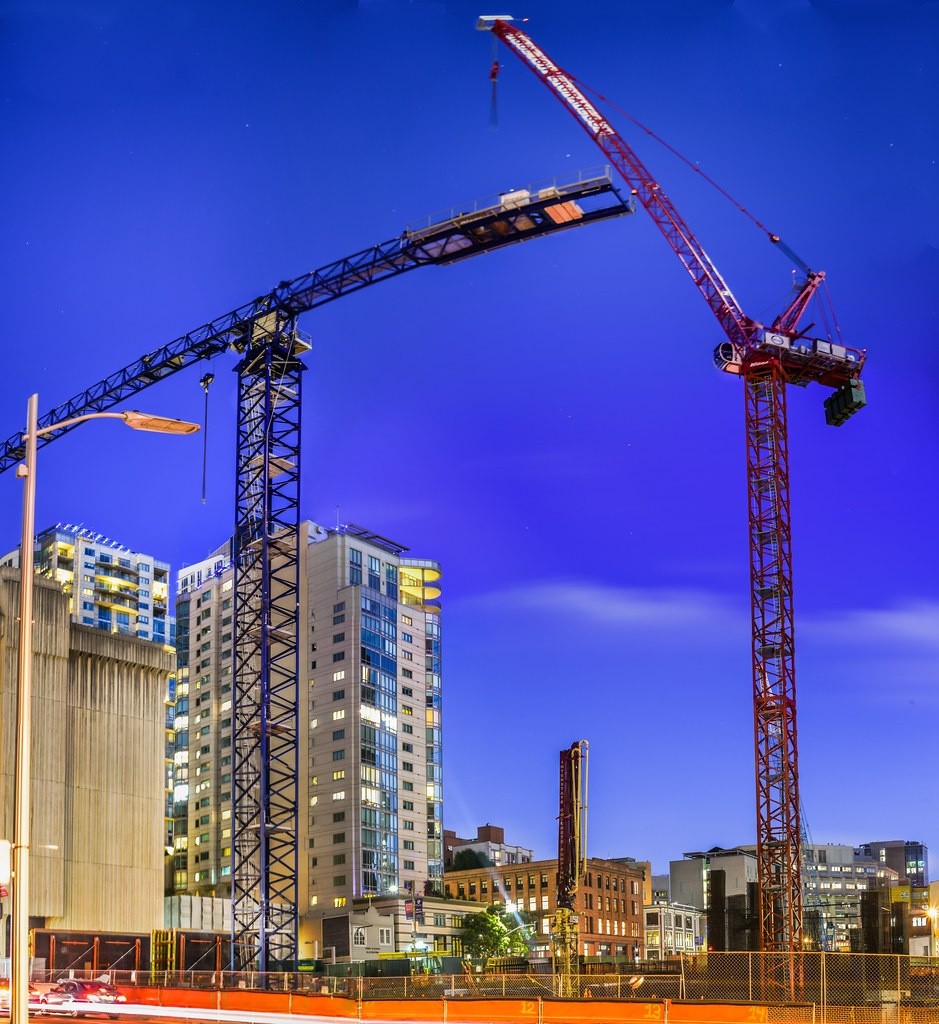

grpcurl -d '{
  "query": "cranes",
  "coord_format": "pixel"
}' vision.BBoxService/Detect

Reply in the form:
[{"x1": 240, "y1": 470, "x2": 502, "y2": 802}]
[
  {"x1": 0, "y1": 165, "x2": 638, "y2": 989},
  {"x1": 477, "y1": 13, "x2": 870, "y2": 1004}
]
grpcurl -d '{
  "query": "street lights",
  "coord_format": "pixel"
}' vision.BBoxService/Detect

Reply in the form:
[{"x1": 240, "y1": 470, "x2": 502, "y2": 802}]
[
  {"x1": 9, "y1": 389, "x2": 205, "y2": 1023},
  {"x1": 389, "y1": 879, "x2": 418, "y2": 976}
]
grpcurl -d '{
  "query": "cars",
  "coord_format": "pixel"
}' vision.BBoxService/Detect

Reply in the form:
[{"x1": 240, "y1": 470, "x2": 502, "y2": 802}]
[
  {"x1": 39, "y1": 979, "x2": 126, "y2": 1019},
  {"x1": 0, "y1": 977, "x2": 42, "y2": 1017}
]
